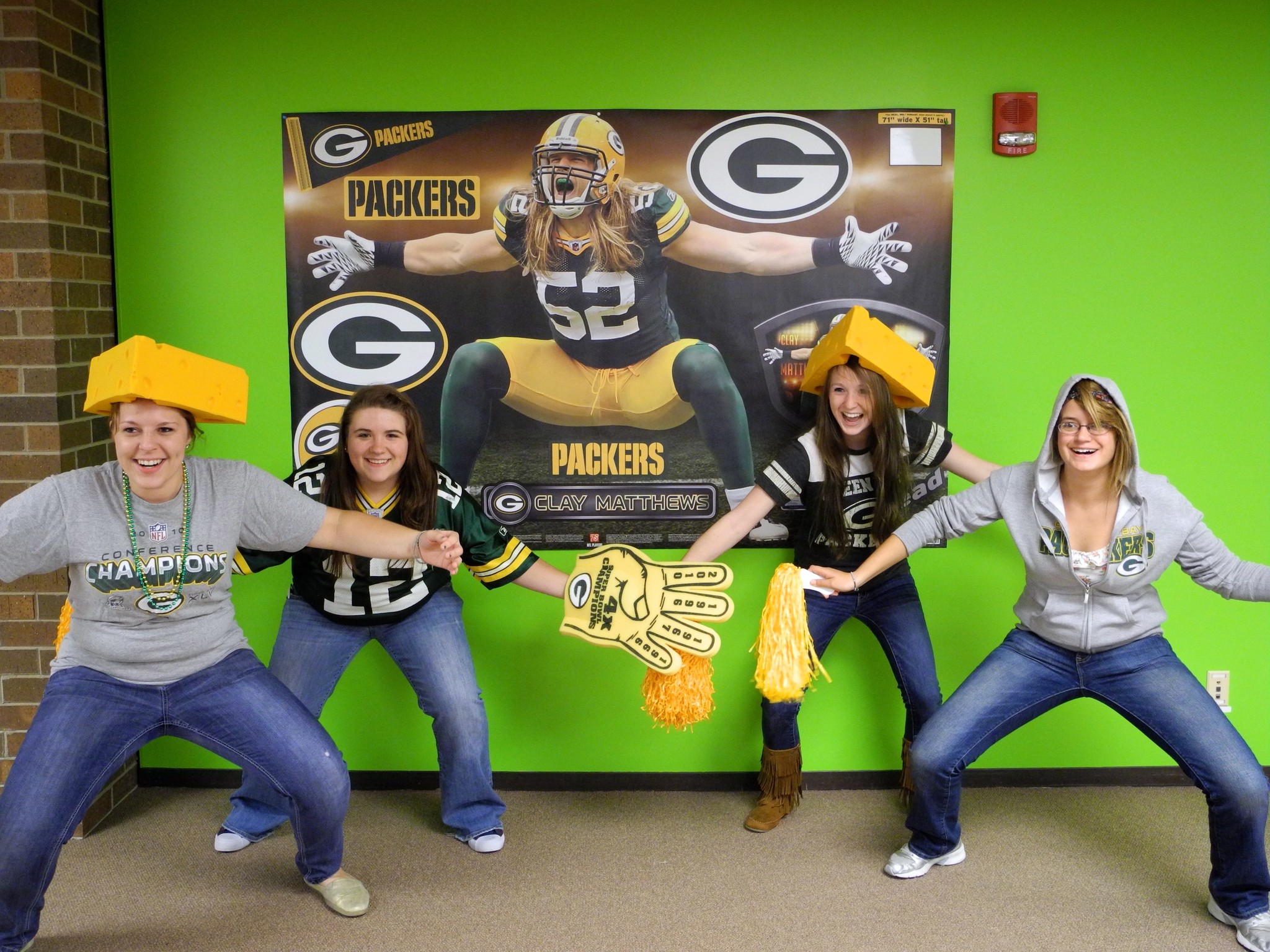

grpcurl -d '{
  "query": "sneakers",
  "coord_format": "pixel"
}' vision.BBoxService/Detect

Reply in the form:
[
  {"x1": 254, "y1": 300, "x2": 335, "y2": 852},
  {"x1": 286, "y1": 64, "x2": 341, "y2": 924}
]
[
  {"x1": 1207, "y1": 892, "x2": 1270, "y2": 951},
  {"x1": 884, "y1": 836, "x2": 966, "y2": 880},
  {"x1": 753, "y1": 518, "x2": 791, "y2": 544}
]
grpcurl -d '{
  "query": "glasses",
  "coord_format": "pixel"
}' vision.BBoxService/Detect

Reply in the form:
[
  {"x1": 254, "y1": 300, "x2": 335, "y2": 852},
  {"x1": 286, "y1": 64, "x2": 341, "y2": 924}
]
[{"x1": 1058, "y1": 421, "x2": 1111, "y2": 436}]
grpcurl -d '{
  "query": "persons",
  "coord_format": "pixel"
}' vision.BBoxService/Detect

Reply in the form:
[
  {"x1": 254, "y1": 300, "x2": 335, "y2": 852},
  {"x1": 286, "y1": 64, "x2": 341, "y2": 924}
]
[
  {"x1": 308, "y1": 113, "x2": 911, "y2": 542},
  {"x1": 213, "y1": 382, "x2": 569, "y2": 852},
  {"x1": 680, "y1": 306, "x2": 1003, "y2": 833},
  {"x1": 808, "y1": 376, "x2": 1270, "y2": 952},
  {"x1": 0, "y1": 335, "x2": 463, "y2": 952}
]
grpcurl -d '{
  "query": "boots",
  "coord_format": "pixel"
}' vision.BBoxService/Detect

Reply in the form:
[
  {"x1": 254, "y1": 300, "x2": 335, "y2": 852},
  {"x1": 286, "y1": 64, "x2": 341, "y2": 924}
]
[
  {"x1": 899, "y1": 737, "x2": 915, "y2": 815},
  {"x1": 743, "y1": 739, "x2": 803, "y2": 833}
]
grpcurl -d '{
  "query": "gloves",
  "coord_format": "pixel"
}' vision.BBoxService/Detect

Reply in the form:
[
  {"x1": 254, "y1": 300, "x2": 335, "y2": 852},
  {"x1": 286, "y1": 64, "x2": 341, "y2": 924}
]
[
  {"x1": 307, "y1": 230, "x2": 409, "y2": 291},
  {"x1": 812, "y1": 214, "x2": 914, "y2": 285},
  {"x1": 916, "y1": 344, "x2": 938, "y2": 362},
  {"x1": 762, "y1": 347, "x2": 792, "y2": 364}
]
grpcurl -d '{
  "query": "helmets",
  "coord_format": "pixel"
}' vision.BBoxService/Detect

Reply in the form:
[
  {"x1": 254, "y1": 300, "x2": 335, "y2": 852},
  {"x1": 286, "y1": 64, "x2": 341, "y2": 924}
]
[
  {"x1": 532, "y1": 112, "x2": 629, "y2": 209},
  {"x1": 830, "y1": 313, "x2": 846, "y2": 327}
]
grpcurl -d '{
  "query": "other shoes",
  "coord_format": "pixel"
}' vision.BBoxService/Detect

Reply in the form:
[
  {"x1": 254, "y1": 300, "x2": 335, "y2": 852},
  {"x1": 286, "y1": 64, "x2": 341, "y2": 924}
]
[
  {"x1": 304, "y1": 867, "x2": 370, "y2": 917},
  {"x1": 214, "y1": 824, "x2": 250, "y2": 852},
  {"x1": 468, "y1": 827, "x2": 505, "y2": 853}
]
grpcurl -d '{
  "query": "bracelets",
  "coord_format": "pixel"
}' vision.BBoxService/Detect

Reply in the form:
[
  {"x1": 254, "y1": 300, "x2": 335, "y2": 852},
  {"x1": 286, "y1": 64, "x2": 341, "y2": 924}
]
[
  {"x1": 413, "y1": 529, "x2": 427, "y2": 564},
  {"x1": 850, "y1": 571, "x2": 859, "y2": 593}
]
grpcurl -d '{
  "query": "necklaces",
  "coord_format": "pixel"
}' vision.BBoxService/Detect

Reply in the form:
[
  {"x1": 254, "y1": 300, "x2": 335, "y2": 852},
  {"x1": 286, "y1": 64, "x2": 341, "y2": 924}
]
[{"x1": 122, "y1": 462, "x2": 190, "y2": 610}]
